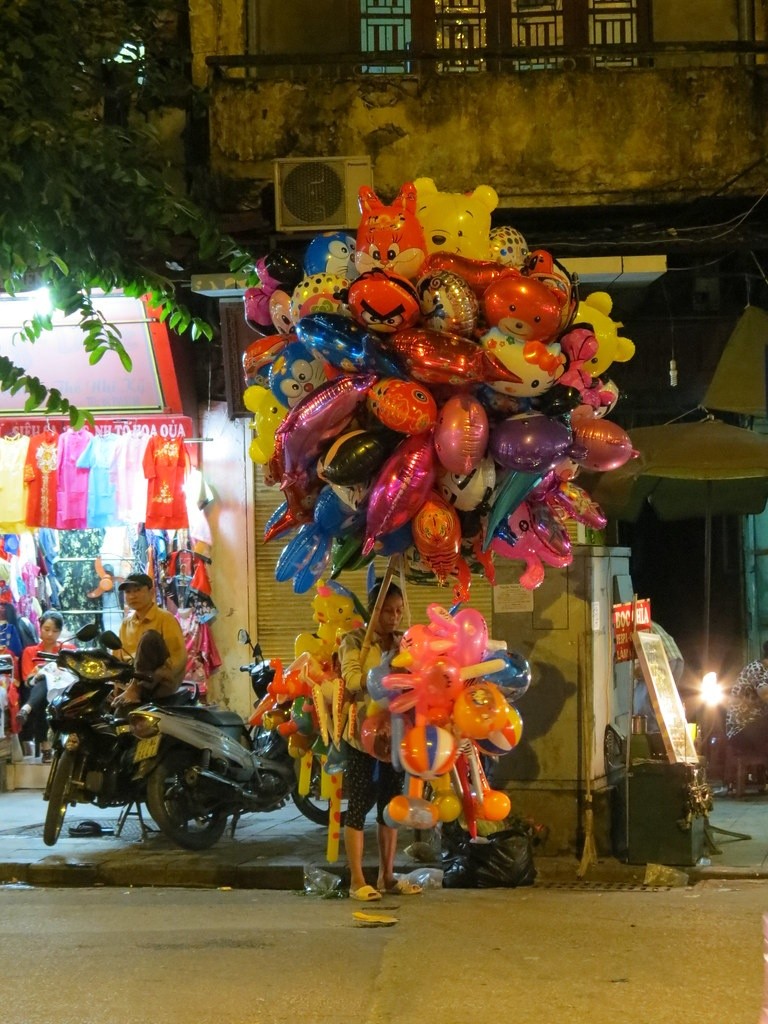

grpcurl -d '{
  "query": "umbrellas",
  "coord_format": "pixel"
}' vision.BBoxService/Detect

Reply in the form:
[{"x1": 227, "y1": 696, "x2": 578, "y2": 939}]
[{"x1": 577, "y1": 305, "x2": 767, "y2": 756}]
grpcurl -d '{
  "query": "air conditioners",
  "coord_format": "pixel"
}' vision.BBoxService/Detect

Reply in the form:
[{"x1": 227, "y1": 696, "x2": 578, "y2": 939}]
[{"x1": 273, "y1": 155, "x2": 374, "y2": 230}]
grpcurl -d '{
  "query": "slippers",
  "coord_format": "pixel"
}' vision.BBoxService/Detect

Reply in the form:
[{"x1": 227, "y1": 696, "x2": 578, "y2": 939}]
[
  {"x1": 347, "y1": 884, "x2": 380, "y2": 900},
  {"x1": 68, "y1": 821, "x2": 113, "y2": 836},
  {"x1": 382, "y1": 881, "x2": 422, "y2": 896}
]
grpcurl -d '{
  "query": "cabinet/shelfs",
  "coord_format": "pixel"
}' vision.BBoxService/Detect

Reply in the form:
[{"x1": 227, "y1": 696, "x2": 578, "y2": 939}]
[{"x1": 54, "y1": 557, "x2": 134, "y2": 615}]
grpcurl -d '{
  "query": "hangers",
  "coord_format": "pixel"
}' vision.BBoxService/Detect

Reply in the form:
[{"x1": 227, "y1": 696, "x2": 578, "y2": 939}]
[{"x1": 4, "y1": 415, "x2": 183, "y2": 438}]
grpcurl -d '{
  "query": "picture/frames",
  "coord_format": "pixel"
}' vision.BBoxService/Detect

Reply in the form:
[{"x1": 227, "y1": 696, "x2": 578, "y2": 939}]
[{"x1": 632, "y1": 631, "x2": 701, "y2": 764}]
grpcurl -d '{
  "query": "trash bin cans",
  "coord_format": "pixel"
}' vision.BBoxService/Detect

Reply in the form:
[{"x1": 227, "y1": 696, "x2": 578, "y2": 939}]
[{"x1": 615, "y1": 762, "x2": 707, "y2": 867}]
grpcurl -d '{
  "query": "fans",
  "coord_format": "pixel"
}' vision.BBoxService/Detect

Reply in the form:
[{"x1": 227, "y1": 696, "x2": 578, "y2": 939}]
[{"x1": 78, "y1": 551, "x2": 135, "y2": 609}]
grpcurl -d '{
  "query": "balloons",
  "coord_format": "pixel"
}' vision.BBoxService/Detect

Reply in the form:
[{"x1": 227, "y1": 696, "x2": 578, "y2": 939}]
[{"x1": 241, "y1": 176, "x2": 640, "y2": 864}]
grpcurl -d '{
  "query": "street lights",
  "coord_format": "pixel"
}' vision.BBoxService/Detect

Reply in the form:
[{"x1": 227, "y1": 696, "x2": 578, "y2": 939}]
[{"x1": 692, "y1": 671, "x2": 726, "y2": 710}]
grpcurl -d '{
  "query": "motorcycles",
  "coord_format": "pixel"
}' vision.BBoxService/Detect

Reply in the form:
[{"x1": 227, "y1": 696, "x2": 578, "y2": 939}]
[
  {"x1": 37, "y1": 626, "x2": 204, "y2": 845},
  {"x1": 113, "y1": 632, "x2": 348, "y2": 850}
]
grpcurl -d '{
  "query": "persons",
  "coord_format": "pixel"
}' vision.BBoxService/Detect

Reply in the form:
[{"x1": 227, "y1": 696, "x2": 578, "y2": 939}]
[
  {"x1": 338, "y1": 577, "x2": 422, "y2": 901},
  {"x1": 726, "y1": 640, "x2": 768, "y2": 754},
  {"x1": 15, "y1": 608, "x2": 77, "y2": 763},
  {"x1": 111, "y1": 574, "x2": 187, "y2": 708}
]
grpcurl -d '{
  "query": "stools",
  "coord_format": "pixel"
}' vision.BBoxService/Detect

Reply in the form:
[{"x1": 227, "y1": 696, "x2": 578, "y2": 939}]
[{"x1": 725, "y1": 755, "x2": 768, "y2": 798}]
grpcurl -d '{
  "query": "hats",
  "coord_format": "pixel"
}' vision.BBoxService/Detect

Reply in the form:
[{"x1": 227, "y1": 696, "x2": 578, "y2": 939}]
[{"x1": 118, "y1": 573, "x2": 153, "y2": 592}]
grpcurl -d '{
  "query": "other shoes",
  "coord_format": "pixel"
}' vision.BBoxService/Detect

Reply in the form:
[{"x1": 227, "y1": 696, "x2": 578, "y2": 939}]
[{"x1": 353, "y1": 911, "x2": 396, "y2": 928}]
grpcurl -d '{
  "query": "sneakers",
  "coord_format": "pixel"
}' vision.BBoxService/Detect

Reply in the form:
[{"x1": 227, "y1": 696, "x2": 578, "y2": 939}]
[
  {"x1": 41, "y1": 750, "x2": 52, "y2": 761},
  {"x1": 17, "y1": 710, "x2": 26, "y2": 718}
]
